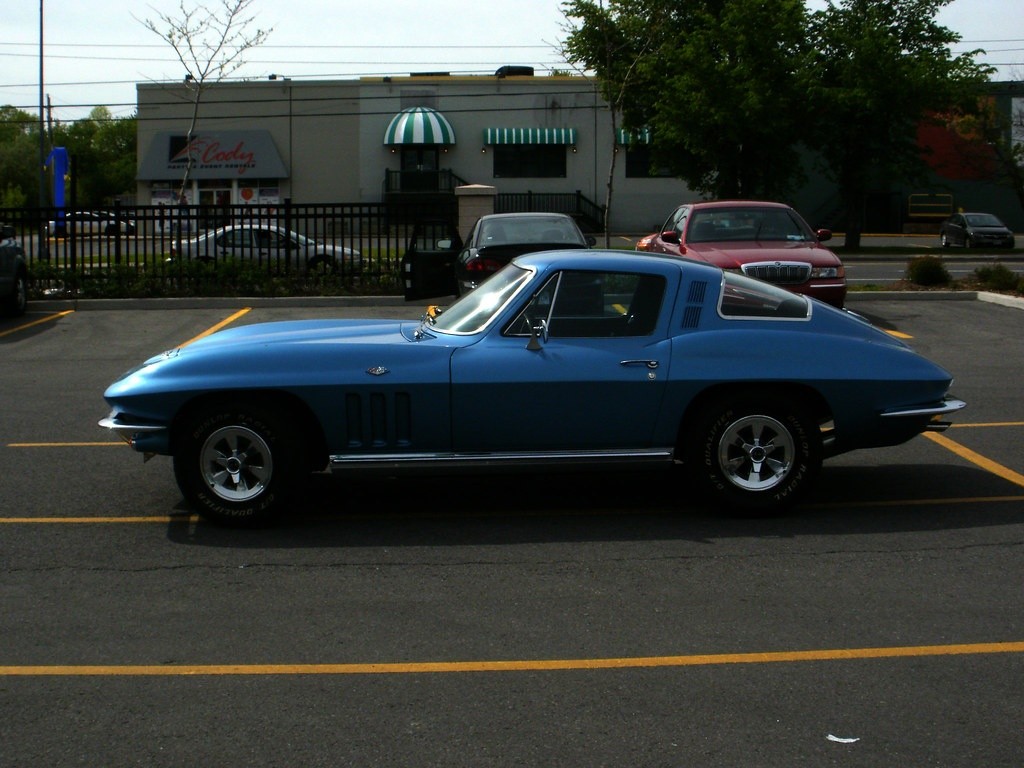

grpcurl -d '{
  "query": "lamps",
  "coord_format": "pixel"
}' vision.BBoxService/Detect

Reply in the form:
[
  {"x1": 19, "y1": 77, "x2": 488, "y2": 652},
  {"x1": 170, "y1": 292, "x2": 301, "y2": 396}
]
[
  {"x1": 482, "y1": 147, "x2": 486, "y2": 153},
  {"x1": 383, "y1": 76, "x2": 390, "y2": 83},
  {"x1": 571, "y1": 147, "x2": 576, "y2": 152},
  {"x1": 269, "y1": 74, "x2": 285, "y2": 80},
  {"x1": 444, "y1": 148, "x2": 447, "y2": 153},
  {"x1": 615, "y1": 148, "x2": 619, "y2": 152},
  {"x1": 391, "y1": 146, "x2": 395, "y2": 153},
  {"x1": 497, "y1": 71, "x2": 505, "y2": 80},
  {"x1": 184, "y1": 74, "x2": 192, "y2": 81}
]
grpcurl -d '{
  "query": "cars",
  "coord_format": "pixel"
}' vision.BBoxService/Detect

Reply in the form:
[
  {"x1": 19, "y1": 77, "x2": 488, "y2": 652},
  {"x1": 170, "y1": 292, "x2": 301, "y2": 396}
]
[
  {"x1": 401, "y1": 212, "x2": 597, "y2": 303},
  {"x1": 97, "y1": 247, "x2": 968, "y2": 531},
  {"x1": 635, "y1": 200, "x2": 846, "y2": 310},
  {"x1": 165, "y1": 225, "x2": 362, "y2": 273},
  {"x1": 46, "y1": 211, "x2": 134, "y2": 236},
  {"x1": 939, "y1": 213, "x2": 1016, "y2": 249},
  {"x1": 0, "y1": 222, "x2": 28, "y2": 318}
]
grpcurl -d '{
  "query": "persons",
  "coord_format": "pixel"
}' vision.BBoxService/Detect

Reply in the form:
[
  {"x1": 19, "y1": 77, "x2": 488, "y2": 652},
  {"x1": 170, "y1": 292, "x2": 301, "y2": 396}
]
[{"x1": 526, "y1": 272, "x2": 592, "y2": 337}]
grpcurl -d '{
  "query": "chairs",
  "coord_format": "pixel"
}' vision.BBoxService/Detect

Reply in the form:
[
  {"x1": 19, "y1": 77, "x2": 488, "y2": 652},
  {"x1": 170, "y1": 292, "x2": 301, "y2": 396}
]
[
  {"x1": 695, "y1": 221, "x2": 716, "y2": 237},
  {"x1": 573, "y1": 273, "x2": 607, "y2": 333}
]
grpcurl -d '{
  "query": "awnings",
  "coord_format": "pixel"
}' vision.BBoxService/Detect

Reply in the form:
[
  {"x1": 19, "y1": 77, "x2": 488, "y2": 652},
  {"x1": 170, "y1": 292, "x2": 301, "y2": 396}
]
[
  {"x1": 483, "y1": 127, "x2": 576, "y2": 144},
  {"x1": 616, "y1": 128, "x2": 652, "y2": 144},
  {"x1": 383, "y1": 106, "x2": 456, "y2": 144}
]
